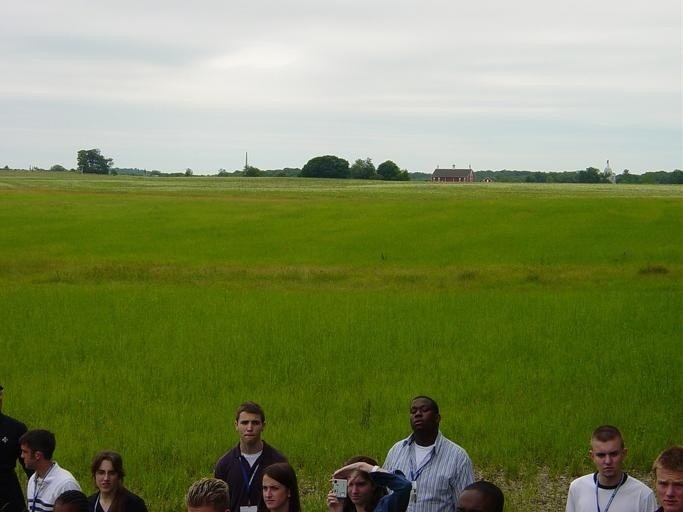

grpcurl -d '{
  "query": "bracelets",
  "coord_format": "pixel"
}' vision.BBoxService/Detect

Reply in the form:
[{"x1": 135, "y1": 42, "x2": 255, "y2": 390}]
[{"x1": 371, "y1": 465, "x2": 380, "y2": 472}]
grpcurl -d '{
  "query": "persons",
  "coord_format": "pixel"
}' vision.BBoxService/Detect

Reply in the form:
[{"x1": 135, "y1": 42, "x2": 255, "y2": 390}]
[
  {"x1": 380, "y1": 396, "x2": 474, "y2": 512},
  {"x1": 0, "y1": 384, "x2": 34, "y2": 511},
  {"x1": 17, "y1": 428, "x2": 82, "y2": 511},
  {"x1": 565, "y1": 424, "x2": 659, "y2": 512},
  {"x1": 85, "y1": 449, "x2": 146, "y2": 511},
  {"x1": 456, "y1": 480, "x2": 504, "y2": 512},
  {"x1": 185, "y1": 476, "x2": 231, "y2": 511},
  {"x1": 52, "y1": 489, "x2": 89, "y2": 511},
  {"x1": 214, "y1": 401, "x2": 289, "y2": 511},
  {"x1": 326, "y1": 454, "x2": 412, "y2": 512},
  {"x1": 651, "y1": 444, "x2": 682, "y2": 512},
  {"x1": 255, "y1": 461, "x2": 302, "y2": 511}
]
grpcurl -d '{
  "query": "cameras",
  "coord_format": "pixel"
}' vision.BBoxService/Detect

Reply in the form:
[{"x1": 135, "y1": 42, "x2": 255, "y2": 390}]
[{"x1": 332, "y1": 479, "x2": 347, "y2": 498}]
